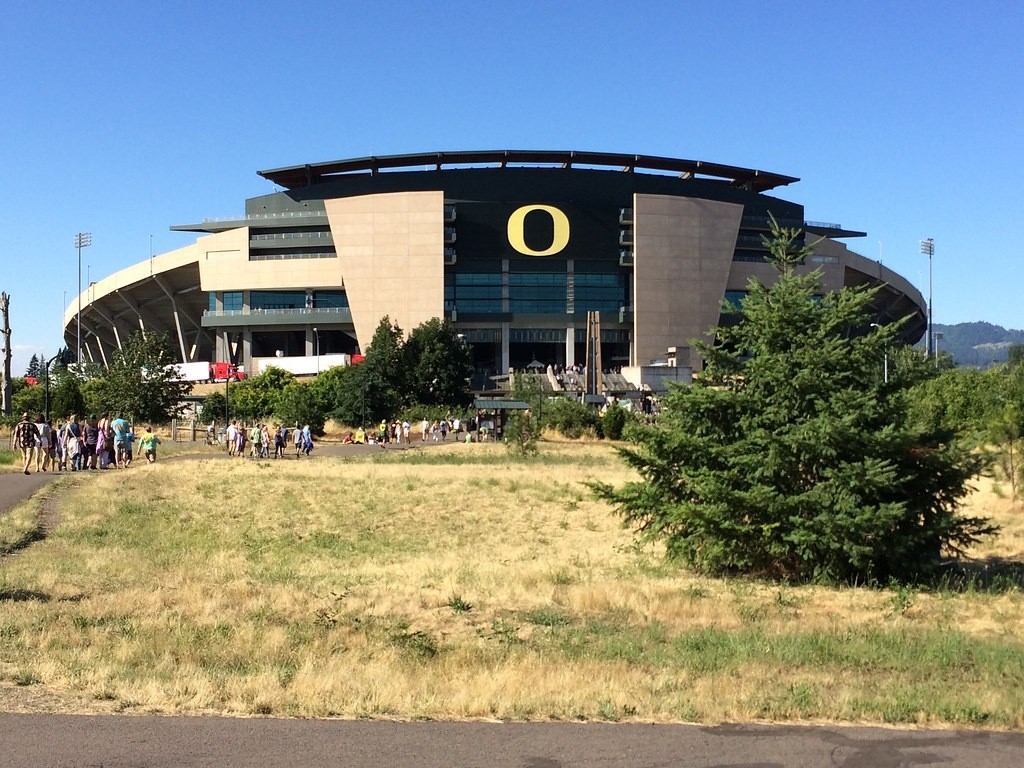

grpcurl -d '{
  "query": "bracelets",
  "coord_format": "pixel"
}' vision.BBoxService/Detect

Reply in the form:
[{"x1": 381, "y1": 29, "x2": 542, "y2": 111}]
[{"x1": 13, "y1": 444, "x2": 16, "y2": 446}]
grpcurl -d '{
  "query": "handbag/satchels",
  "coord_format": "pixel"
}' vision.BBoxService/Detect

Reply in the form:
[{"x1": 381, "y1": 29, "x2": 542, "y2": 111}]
[
  {"x1": 103, "y1": 437, "x2": 113, "y2": 452},
  {"x1": 78, "y1": 440, "x2": 85, "y2": 449}
]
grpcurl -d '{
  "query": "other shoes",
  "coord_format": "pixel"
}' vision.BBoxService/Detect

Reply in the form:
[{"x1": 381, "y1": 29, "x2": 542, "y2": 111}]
[{"x1": 24, "y1": 471, "x2": 31, "y2": 475}]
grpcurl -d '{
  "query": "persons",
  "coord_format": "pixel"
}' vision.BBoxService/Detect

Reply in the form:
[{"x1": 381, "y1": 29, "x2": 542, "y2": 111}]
[
  {"x1": 554, "y1": 363, "x2": 586, "y2": 388},
  {"x1": 343, "y1": 419, "x2": 410, "y2": 448},
  {"x1": 479, "y1": 427, "x2": 488, "y2": 442},
  {"x1": 13, "y1": 412, "x2": 162, "y2": 475},
  {"x1": 226, "y1": 420, "x2": 314, "y2": 460},
  {"x1": 463, "y1": 431, "x2": 473, "y2": 443},
  {"x1": 604, "y1": 365, "x2": 624, "y2": 374},
  {"x1": 422, "y1": 411, "x2": 461, "y2": 441}
]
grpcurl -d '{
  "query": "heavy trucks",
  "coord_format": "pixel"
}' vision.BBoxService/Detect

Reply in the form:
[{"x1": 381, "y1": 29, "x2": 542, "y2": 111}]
[
  {"x1": 260, "y1": 355, "x2": 367, "y2": 380},
  {"x1": 141, "y1": 361, "x2": 248, "y2": 388}
]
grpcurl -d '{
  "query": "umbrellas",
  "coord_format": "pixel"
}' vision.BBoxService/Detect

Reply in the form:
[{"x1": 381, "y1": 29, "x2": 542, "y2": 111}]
[{"x1": 527, "y1": 361, "x2": 544, "y2": 373}]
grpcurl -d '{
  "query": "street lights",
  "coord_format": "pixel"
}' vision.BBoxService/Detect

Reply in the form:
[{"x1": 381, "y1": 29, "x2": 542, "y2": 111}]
[
  {"x1": 932, "y1": 331, "x2": 947, "y2": 369},
  {"x1": 314, "y1": 328, "x2": 320, "y2": 378},
  {"x1": 361, "y1": 378, "x2": 373, "y2": 432},
  {"x1": 74, "y1": 231, "x2": 92, "y2": 373},
  {"x1": 919, "y1": 238, "x2": 935, "y2": 359},
  {"x1": 227, "y1": 364, "x2": 239, "y2": 433},
  {"x1": 870, "y1": 322, "x2": 894, "y2": 384},
  {"x1": 44, "y1": 353, "x2": 65, "y2": 423}
]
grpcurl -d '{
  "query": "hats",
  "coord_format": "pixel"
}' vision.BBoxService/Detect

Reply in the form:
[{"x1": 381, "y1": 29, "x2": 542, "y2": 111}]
[
  {"x1": 47, "y1": 420, "x2": 52, "y2": 427},
  {"x1": 90, "y1": 414, "x2": 98, "y2": 421},
  {"x1": 22, "y1": 412, "x2": 31, "y2": 418}
]
